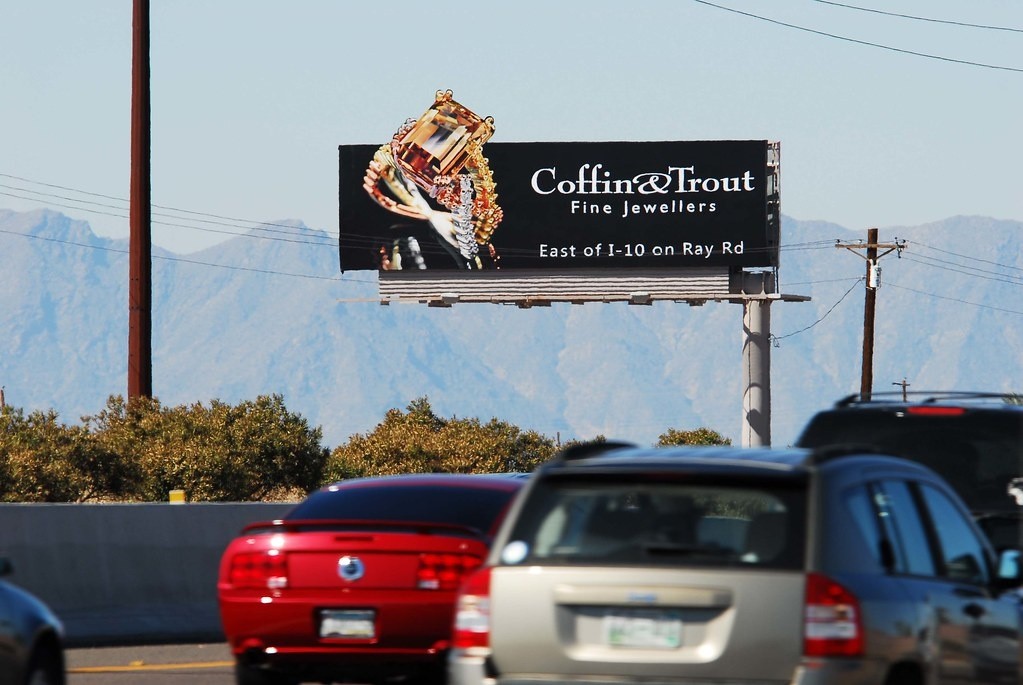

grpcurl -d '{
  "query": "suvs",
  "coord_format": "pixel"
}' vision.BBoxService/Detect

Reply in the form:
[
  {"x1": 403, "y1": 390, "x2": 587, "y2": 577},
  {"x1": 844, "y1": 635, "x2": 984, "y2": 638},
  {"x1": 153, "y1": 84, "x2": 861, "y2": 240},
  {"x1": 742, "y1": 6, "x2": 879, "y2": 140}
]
[
  {"x1": 791, "y1": 386, "x2": 1023, "y2": 591},
  {"x1": 443, "y1": 439, "x2": 1023, "y2": 685}
]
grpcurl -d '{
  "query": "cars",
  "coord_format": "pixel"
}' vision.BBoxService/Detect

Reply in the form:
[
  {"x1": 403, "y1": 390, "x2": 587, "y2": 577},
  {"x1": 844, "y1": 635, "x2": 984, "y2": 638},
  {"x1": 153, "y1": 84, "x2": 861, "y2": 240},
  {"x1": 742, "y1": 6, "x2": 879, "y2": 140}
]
[
  {"x1": 0, "y1": 560, "x2": 67, "y2": 685},
  {"x1": 216, "y1": 472, "x2": 536, "y2": 685}
]
